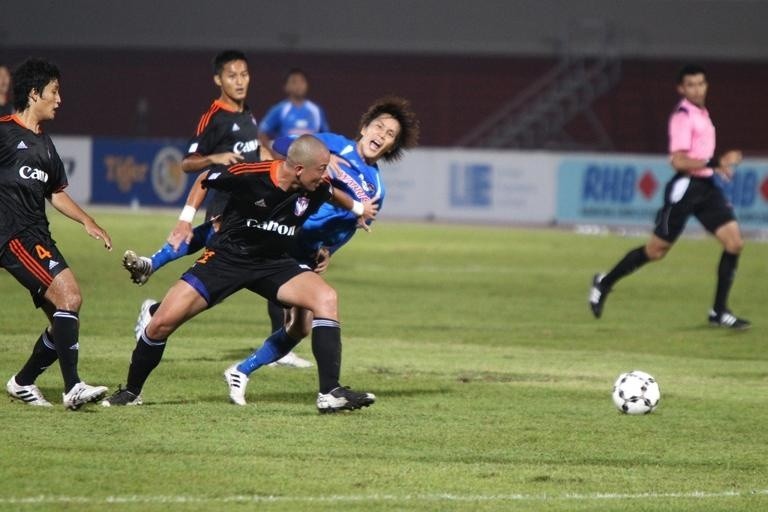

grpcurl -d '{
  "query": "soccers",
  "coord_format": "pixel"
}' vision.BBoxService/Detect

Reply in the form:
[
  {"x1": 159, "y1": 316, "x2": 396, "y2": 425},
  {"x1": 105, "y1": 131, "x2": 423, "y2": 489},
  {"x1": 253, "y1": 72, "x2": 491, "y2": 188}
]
[{"x1": 612, "y1": 370, "x2": 660, "y2": 414}]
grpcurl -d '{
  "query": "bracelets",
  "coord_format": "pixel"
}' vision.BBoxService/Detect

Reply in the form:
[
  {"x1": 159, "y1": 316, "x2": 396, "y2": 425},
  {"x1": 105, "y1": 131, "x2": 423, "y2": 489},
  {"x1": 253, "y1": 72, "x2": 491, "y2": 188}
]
[{"x1": 706, "y1": 156, "x2": 721, "y2": 168}]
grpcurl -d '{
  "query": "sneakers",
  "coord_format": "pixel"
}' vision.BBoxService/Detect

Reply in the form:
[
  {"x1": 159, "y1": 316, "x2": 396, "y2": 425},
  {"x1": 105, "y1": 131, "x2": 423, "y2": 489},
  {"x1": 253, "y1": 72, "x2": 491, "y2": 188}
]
[
  {"x1": 223, "y1": 350, "x2": 312, "y2": 407},
  {"x1": 318, "y1": 384, "x2": 375, "y2": 413},
  {"x1": 708, "y1": 306, "x2": 752, "y2": 328},
  {"x1": 6, "y1": 375, "x2": 143, "y2": 410},
  {"x1": 122, "y1": 251, "x2": 153, "y2": 285},
  {"x1": 590, "y1": 273, "x2": 607, "y2": 318},
  {"x1": 135, "y1": 299, "x2": 156, "y2": 343}
]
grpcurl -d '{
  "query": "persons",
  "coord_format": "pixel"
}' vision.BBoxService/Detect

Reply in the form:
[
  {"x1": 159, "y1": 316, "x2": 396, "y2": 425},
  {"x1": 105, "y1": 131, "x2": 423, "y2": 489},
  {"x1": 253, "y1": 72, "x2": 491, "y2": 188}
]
[
  {"x1": 1, "y1": 62, "x2": 15, "y2": 115},
  {"x1": 102, "y1": 133, "x2": 376, "y2": 411},
  {"x1": 590, "y1": 65, "x2": 752, "y2": 331},
  {"x1": 0, "y1": 56, "x2": 109, "y2": 409}
]
